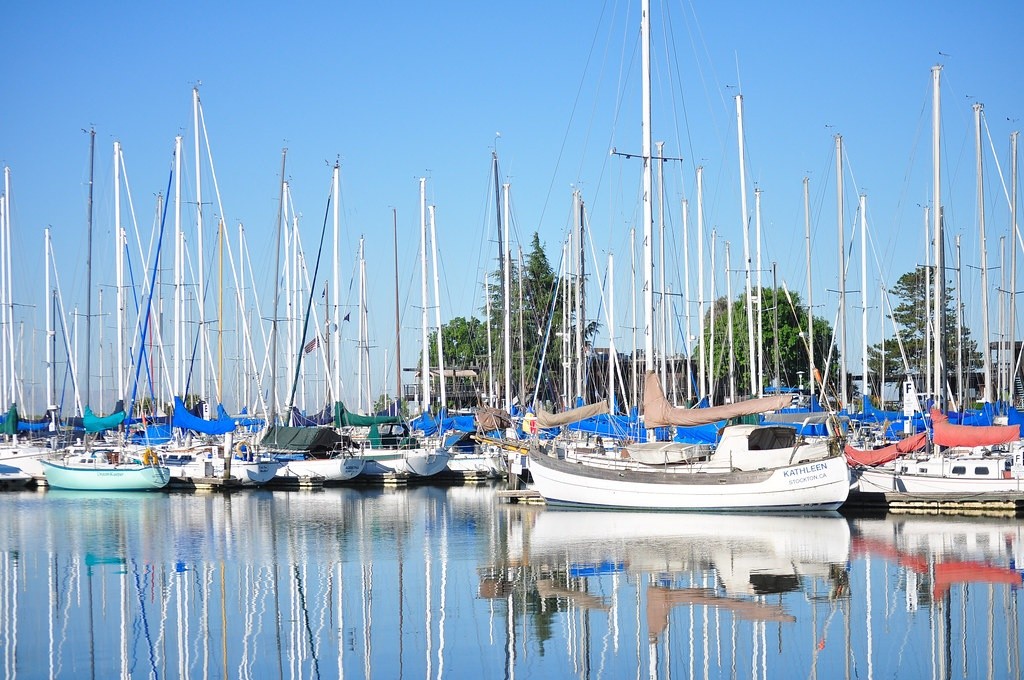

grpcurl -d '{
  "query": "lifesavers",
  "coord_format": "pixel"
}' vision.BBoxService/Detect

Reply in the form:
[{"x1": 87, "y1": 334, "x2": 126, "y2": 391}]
[
  {"x1": 235, "y1": 440, "x2": 251, "y2": 458},
  {"x1": 143, "y1": 448, "x2": 158, "y2": 467}
]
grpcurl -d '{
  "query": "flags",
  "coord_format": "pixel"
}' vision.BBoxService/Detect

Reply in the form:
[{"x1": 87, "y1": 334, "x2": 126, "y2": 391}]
[{"x1": 305, "y1": 336, "x2": 320, "y2": 353}]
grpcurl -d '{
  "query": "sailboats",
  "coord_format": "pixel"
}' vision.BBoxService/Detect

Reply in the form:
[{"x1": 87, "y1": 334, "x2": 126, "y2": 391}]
[
  {"x1": 0, "y1": 0, "x2": 1024, "y2": 501},
  {"x1": 526, "y1": 142, "x2": 850, "y2": 510}
]
